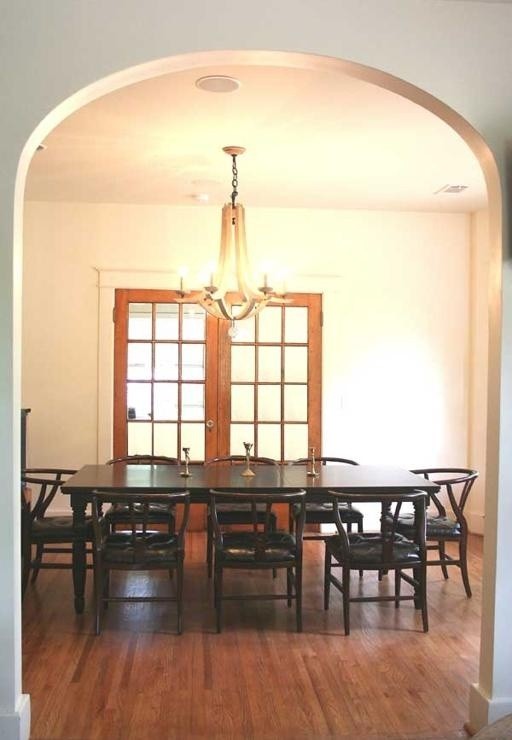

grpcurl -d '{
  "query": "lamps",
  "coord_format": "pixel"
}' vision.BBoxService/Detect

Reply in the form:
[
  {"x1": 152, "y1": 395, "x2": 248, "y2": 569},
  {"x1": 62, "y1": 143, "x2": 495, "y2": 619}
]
[{"x1": 172, "y1": 146, "x2": 296, "y2": 321}]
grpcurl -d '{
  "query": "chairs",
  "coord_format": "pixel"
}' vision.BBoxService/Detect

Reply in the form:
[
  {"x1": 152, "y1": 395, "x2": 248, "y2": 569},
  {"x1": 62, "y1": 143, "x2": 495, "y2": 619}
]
[
  {"x1": 208, "y1": 487, "x2": 307, "y2": 634},
  {"x1": 322, "y1": 488, "x2": 431, "y2": 634},
  {"x1": 291, "y1": 455, "x2": 364, "y2": 577},
  {"x1": 88, "y1": 488, "x2": 192, "y2": 631},
  {"x1": 22, "y1": 467, "x2": 100, "y2": 602},
  {"x1": 205, "y1": 454, "x2": 280, "y2": 580},
  {"x1": 103, "y1": 454, "x2": 182, "y2": 580},
  {"x1": 378, "y1": 465, "x2": 477, "y2": 600}
]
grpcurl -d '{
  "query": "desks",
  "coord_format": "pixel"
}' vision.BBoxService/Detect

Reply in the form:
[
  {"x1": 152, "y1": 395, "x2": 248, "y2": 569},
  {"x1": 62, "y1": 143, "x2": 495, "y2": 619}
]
[{"x1": 58, "y1": 464, "x2": 443, "y2": 617}]
